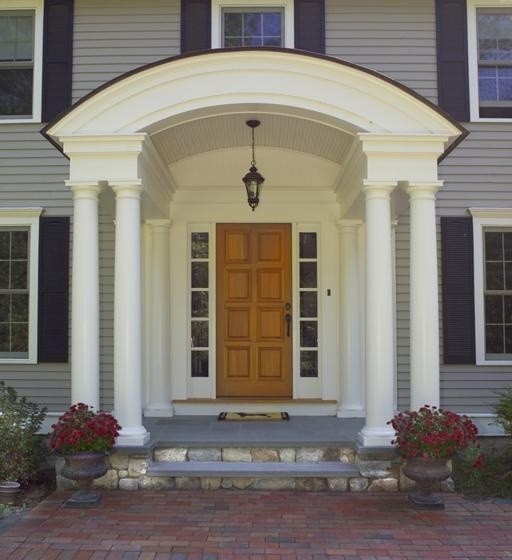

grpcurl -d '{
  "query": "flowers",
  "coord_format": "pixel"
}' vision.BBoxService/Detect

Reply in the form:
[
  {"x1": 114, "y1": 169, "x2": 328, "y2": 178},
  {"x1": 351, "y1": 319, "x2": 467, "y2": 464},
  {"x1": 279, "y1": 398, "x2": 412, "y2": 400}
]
[
  {"x1": 387, "y1": 404, "x2": 488, "y2": 470},
  {"x1": 48, "y1": 402, "x2": 121, "y2": 455}
]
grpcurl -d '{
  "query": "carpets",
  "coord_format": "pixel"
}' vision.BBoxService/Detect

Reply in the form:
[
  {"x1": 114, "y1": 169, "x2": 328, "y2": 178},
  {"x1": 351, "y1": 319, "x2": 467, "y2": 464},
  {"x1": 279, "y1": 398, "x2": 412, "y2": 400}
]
[{"x1": 219, "y1": 411, "x2": 291, "y2": 421}]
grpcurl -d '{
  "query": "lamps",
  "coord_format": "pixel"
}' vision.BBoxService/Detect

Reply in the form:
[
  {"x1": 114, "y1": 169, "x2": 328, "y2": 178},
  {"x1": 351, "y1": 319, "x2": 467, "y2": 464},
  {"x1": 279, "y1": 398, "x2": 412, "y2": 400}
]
[{"x1": 242, "y1": 119, "x2": 265, "y2": 210}]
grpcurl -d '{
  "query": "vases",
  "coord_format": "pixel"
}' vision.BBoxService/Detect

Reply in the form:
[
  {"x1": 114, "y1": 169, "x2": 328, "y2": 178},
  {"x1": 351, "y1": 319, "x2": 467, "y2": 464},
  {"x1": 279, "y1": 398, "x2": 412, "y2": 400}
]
[
  {"x1": 59, "y1": 451, "x2": 110, "y2": 506},
  {"x1": 399, "y1": 457, "x2": 454, "y2": 510},
  {"x1": 0, "y1": 480, "x2": 21, "y2": 507}
]
